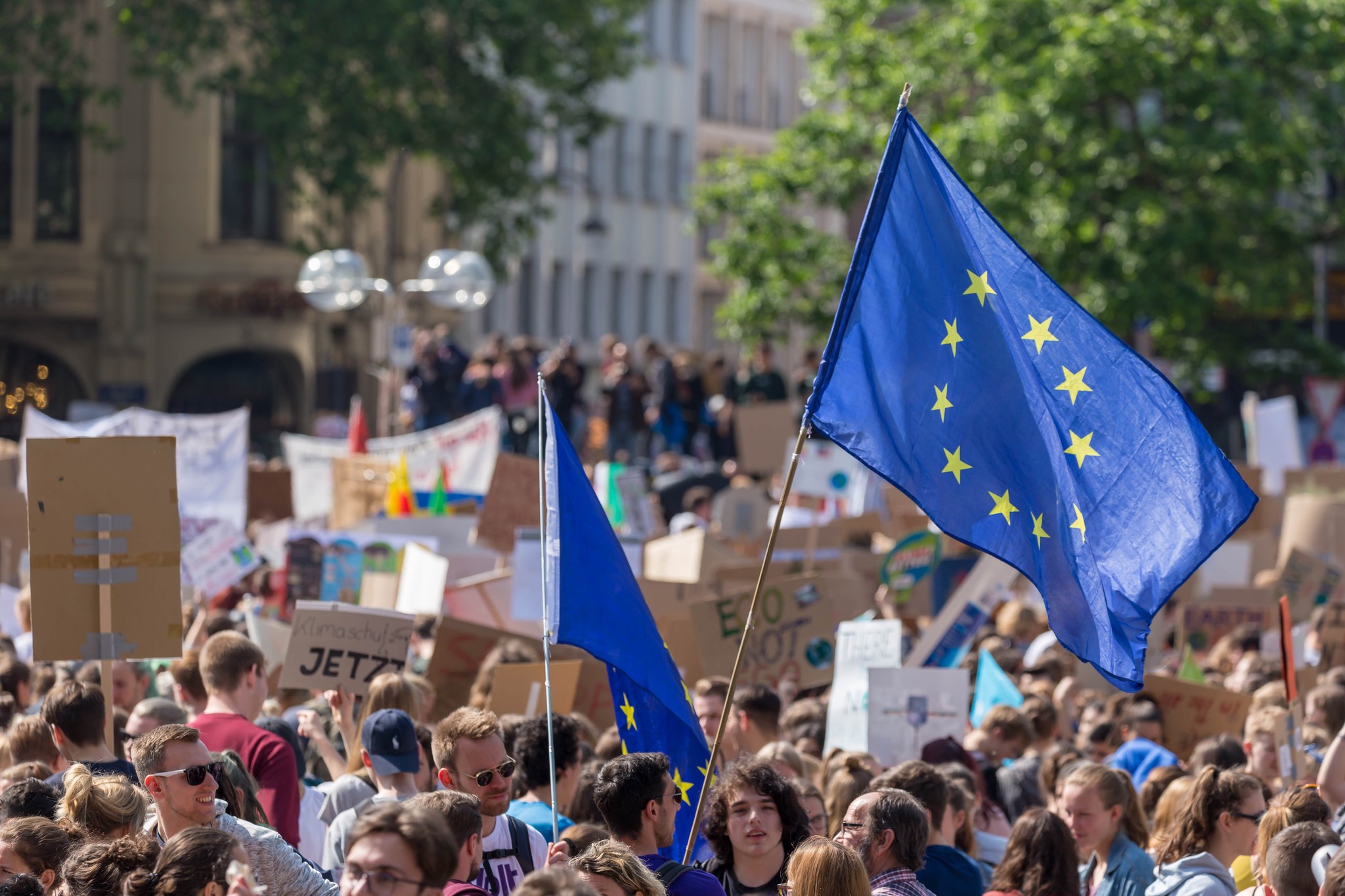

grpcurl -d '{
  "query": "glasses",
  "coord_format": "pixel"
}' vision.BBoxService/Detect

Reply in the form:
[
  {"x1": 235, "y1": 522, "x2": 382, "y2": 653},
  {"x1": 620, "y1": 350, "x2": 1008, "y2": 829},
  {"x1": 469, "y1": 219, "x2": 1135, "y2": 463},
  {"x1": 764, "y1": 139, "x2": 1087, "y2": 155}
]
[
  {"x1": 1302, "y1": 783, "x2": 1321, "y2": 796},
  {"x1": 341, "y1": 862, "x2": 422, "y2": 896},
  {"x1": 841, "y1": 822, "x2": 864, "y2": 836},
  {"x1": 1214, "y1": 811, "x2": 1267, "y2": 826},
  {"x1": 118, "y1": 727, "x2": 141, "y2": 745},
  {"x1": 448, "y1": 756, "x2": 516, "y2": 788},
  {"x1": 153, "y1": 761, "x2": 225, "y2": 786},
  {"x1": 655, "y1": 786, "x2": 682, "y2": 804}
]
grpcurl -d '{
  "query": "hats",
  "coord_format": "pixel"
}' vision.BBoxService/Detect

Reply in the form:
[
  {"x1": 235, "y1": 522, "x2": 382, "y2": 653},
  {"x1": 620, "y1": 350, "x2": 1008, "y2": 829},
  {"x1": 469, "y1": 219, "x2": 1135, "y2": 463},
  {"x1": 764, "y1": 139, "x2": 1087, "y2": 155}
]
[{"x1": 361, "y1": 708, "x2": 420, "y2": 776}]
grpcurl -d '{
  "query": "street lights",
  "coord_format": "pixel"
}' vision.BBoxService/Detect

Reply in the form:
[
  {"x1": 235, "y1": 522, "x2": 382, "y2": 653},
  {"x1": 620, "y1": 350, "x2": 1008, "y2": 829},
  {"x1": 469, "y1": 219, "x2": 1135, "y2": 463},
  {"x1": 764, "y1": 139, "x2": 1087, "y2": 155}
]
[{"x1": 292, "y1": 246, "x2": 498, "y2": 440}]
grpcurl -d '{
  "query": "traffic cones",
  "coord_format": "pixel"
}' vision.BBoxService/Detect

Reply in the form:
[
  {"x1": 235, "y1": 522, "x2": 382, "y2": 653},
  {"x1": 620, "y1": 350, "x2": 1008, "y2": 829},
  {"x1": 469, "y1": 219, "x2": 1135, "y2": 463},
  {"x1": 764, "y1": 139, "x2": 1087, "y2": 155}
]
[{"x1": 345, "y1": 406, "x2": 369, "y2": 451}]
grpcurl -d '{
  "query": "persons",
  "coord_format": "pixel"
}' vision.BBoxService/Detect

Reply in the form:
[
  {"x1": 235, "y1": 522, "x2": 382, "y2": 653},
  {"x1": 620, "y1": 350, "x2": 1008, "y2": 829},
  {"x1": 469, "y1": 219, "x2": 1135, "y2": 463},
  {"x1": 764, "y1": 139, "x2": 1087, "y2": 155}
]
[
  {"x1": 0, "y1": 554, "x2": 1345, "y2": 896},
  {"x1": 398, "y1": 320, "x2": 822, "y2": 530}
]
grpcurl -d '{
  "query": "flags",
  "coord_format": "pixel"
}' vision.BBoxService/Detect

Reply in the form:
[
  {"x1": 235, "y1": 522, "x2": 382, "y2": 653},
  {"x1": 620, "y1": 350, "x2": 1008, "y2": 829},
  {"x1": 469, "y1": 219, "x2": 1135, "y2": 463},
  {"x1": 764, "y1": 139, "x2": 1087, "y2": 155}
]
[
  {"x1": 805, "y1": 108, "x2": 1258, "y2": 693},
  {"x1": 540, "y1": 383, "x2": 715, "y2": 866}
]
